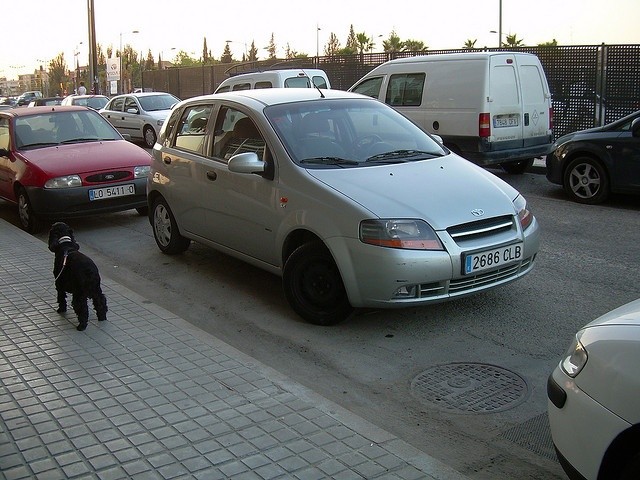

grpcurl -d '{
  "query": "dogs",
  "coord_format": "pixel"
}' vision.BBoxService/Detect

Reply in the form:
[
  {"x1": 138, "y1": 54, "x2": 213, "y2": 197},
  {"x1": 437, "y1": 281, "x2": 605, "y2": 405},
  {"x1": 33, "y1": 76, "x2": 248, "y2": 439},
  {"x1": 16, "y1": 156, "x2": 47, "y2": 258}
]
[{"x1": 48, "y1": 222, "x2": 108, "y2": 331}]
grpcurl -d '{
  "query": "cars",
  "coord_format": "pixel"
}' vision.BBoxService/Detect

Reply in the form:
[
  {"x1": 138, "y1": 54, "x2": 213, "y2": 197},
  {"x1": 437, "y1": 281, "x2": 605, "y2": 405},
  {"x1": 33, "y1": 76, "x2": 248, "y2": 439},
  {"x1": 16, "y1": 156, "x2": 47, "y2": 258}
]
[
  {"x1": 0, "y1": 104, "x2": 152, "y2": 234},
  {"x1": 61, "y1": 94, "x2": 110, "y2": 111},
  {"x1": 545, "y1": 112, "x2": 640, "y2": 204},
  {"x1": 545, "y1": 299, "x2": 639, "y2": 479},
  {"x1": 99, "y1": 91, "x2": 182, "y2": 149},
  {"x1": 28, "y1": 98, "x2": 62, "y2": 106},
  {"x1": 16, "y1": 91, "x2": 41, "y2": 106},
  {"x1": 144, "y1": 87, "x2": 540, "y2": 325}
]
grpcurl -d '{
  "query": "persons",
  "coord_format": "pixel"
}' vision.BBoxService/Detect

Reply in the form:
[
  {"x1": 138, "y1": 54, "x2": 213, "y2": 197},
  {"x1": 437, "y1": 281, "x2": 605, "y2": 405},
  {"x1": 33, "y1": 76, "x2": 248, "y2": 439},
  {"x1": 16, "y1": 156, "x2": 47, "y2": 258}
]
[{"x1": 78, "y1": 82, "x2": 86, "y2": 95}]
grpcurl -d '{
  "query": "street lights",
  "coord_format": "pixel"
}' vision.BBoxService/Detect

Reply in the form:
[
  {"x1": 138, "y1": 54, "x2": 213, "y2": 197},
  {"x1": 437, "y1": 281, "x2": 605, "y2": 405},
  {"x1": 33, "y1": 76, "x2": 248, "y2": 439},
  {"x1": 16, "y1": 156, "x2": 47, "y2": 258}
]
[{"x1": 119, "y1": 30, "x2": 139, "y2": 94}]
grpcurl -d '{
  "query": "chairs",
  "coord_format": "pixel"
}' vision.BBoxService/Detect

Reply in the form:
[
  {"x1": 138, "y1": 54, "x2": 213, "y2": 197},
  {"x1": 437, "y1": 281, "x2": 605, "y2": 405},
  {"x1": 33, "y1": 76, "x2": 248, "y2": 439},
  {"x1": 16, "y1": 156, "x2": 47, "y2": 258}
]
[
  {"x1": 7, "y1": 125, "x2": 35, "y2": 151},
  {"x1": 222, "y1": 118, "x2": 272, "y2": 177},
  {"x1": 297, "y1": 113, "x2": 345, "y2": 159}
]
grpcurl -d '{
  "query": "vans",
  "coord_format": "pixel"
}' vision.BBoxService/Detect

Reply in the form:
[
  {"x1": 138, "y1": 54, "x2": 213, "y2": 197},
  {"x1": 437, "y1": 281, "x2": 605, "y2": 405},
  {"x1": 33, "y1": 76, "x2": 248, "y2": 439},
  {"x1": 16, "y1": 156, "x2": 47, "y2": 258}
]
[
  {"x1": 346, "y1": 51, "x2": 553, "y2": 173},
  {"x1": 213, "y1": 67, "x2": 331, "y2": 94}
]
